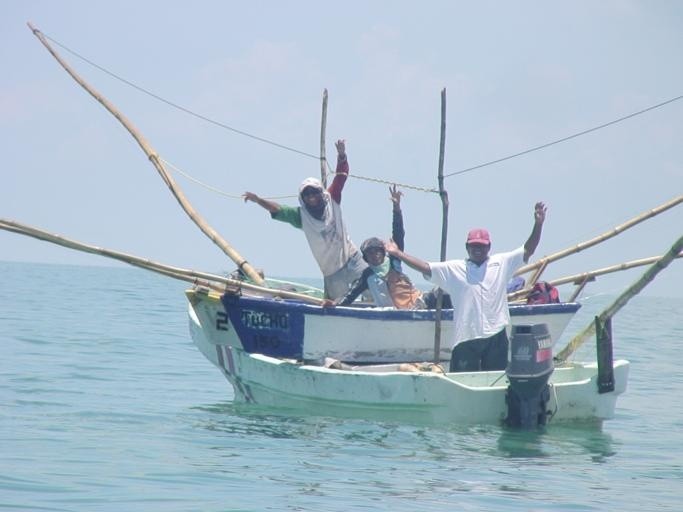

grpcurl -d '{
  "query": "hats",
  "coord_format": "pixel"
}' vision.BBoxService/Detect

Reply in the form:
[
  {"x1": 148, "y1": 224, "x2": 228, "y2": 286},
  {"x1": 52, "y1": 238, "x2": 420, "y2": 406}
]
[
  {"x1": 299, "y1": 176, "x2": 324, "y2": 196},
  {"x1": 466, "y1": 228, "x2": 491, "y2": 245},
  {"x1": 360, "y1": 237, "x2": 386, "y2": 254}
]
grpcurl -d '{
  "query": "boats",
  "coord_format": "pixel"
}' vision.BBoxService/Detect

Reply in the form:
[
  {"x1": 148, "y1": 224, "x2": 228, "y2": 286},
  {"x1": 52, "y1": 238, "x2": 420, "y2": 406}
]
[
  {"x1": 186, "y1": 278, "x2": 583, "y2": 372},
  {"x1": 176, "y1": 283, "x2": 633, "y2": 428}
]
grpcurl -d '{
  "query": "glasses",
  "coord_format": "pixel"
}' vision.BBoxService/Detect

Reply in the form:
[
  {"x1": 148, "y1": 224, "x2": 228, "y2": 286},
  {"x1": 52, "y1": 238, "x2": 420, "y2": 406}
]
[{"x1": 303, "y1": 188, "x2": 321, "y2": 195}]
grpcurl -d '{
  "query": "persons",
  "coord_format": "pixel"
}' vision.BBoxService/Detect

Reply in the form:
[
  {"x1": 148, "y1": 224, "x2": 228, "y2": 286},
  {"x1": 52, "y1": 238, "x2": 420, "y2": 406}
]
[
  {"x1": 321, "y1": 183, "x2": 424, "y2": 310},
  {"x1": 242, "y1": 139, "x2": 369, "y2": 300},
  {"x1": 381, "y1": 202, "x2": 547, "y2": 373}
]
